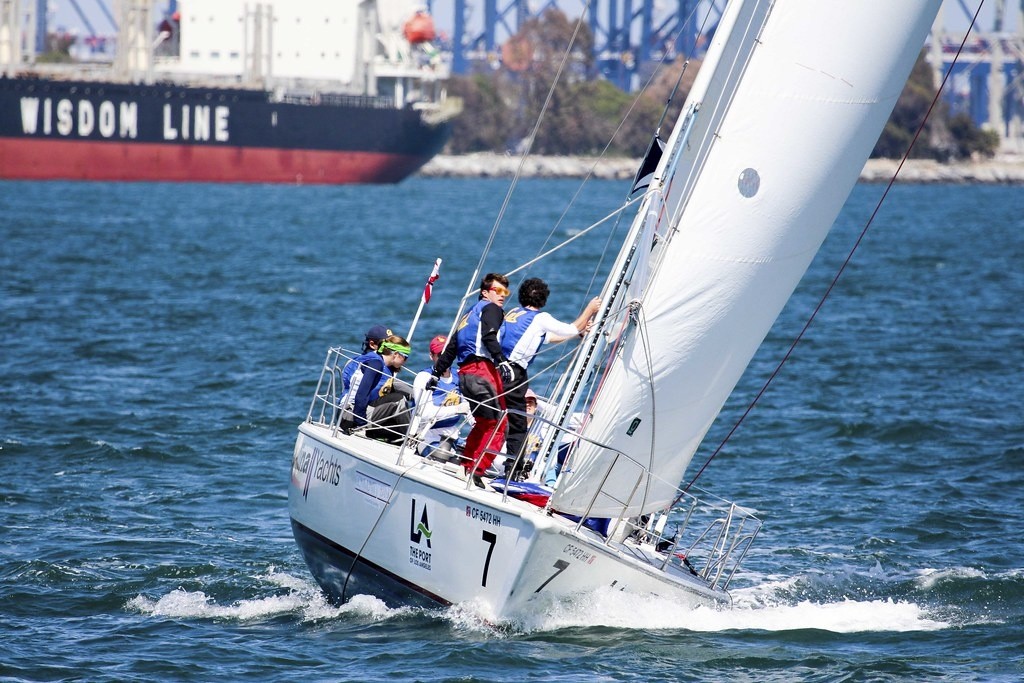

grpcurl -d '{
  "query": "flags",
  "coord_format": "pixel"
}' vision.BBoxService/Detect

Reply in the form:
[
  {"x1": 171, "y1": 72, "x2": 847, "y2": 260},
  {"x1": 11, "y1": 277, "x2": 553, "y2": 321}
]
[
  {"x1": 633, "y1": 137, "x2": 668, "y2": 192},
  {"x1": 424, "y1": 273, "x2": 439, "y2": 303}
]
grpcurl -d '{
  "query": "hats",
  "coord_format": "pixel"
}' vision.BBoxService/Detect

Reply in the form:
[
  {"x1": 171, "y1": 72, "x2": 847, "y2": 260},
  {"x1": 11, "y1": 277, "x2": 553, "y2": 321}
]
[
  {"x1": 365, "y1": 327, "x2": 390, "y2": 338},
  {"x1": 430, "y1": 336, "x2": 448, "y2": 354},
  {"x1": 524, "y1": 388, "x2": 537, "y2": 404}
]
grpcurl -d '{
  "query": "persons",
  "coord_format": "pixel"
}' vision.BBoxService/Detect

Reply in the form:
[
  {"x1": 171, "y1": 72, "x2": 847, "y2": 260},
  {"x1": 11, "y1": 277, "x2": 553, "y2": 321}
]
[
  {"x1": 339, "y1": 326, "x2": 477, "y2": 463},
  {"x1": 493, "y1": 279, "x2": 601, "y2": 482},
  {"x1": 425, "y1": 273, "x2": 515, "y2": 493}
]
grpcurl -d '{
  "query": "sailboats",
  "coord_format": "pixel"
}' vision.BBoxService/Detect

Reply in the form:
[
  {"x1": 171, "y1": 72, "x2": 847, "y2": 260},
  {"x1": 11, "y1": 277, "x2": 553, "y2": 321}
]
[{"x1": 293, "y1": 0, "x2": 984, "y2": 626}]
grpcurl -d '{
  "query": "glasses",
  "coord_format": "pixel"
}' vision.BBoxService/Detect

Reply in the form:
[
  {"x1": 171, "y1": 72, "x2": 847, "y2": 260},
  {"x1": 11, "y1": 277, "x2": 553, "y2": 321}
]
[
  {"x1": 373, "y1": 339, "x2": 387, "y2": 349},
  {"x1": 391, "y1": 351, "x2": 409, "y2": 361},
  {"x1": 432, "y1": 351, "x2": 441, "y2": 358},
  {"x1": 489, "y1": 286, "x2": 511, "y2": 296}
]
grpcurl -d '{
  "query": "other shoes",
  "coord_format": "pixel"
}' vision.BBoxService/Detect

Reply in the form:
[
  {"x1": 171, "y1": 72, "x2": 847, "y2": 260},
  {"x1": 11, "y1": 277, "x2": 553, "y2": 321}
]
[{"x1": 473, "y1": 474, "x2": 496, "y2": 493}]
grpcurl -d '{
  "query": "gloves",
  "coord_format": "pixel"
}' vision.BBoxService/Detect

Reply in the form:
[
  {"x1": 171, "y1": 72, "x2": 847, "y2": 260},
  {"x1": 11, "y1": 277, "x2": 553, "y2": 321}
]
[
  {"x1": 426, "y1": 378, "x2": 437, "y2": 391},
  {"x1": 499, "y1": 361, "x2": 515, "y2": 384},
  {"x1": 353, "y1": 426, "x2": 368, "y2": 439},
  {"x1": 440, "y1": 388, "x2": 461, "y2": 406}
]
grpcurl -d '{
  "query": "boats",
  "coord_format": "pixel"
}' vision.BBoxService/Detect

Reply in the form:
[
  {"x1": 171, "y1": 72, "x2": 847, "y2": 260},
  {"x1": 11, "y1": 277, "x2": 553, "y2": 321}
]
[{"x1": 0, "y1": 0, "x2": 465, "y2": 185}]
